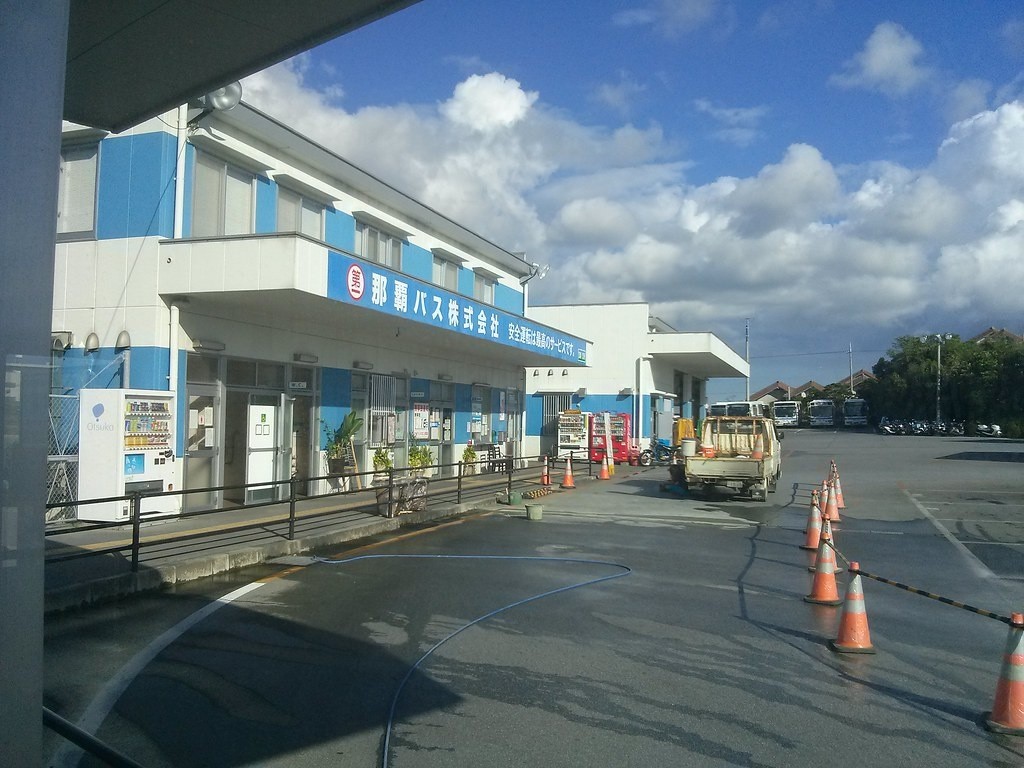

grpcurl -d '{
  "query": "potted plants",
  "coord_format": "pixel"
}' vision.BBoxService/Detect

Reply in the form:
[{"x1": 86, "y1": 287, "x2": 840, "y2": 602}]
[
  {"x1": 321, "y1": 410, "x2": 364, "y2": 474},
  {"x1": 373, "y1": 446, "x2": 392, "y2": 481},
  {"x1": 408, "y1": 444, "x2": 435, "y2": 478},
  {"x1": 463, "y1": 447, "x2": 477, "y2": 465}
]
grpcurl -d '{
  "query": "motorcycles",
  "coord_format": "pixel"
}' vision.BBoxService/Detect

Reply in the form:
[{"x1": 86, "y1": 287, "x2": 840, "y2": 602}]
[
  {"x1": 640, "y1": 433, "x2": 681, "y2": 469},
  {"x1": 877, "y1": 416, "x2": 948, "y2": 435}
]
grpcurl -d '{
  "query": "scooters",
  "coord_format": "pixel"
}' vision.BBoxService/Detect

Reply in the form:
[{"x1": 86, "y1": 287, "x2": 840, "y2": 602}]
[
  {"x1": 950, "y1": 417, "x2": 967, "y2": 435},
  {"x1": 975, "y1": 419, "x2": 1004, "y2": 437}
]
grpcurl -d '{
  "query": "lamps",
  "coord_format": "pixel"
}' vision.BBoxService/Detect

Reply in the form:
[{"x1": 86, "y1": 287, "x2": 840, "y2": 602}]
[
  {"x1": 533, "y1": 262, "x2": 550, "y2": 280},
  {"x1": 207, "y1": 80, "x2": 242, "y2": 110}
]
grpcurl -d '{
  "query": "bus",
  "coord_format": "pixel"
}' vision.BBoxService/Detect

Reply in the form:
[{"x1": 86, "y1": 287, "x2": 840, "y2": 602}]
[
  {"x1": 769, "y1": 399, "x2": 802, "y2": 429},
  {"x1": 806, "y1": 398, "x2": 836, "y2": 426},
  {"x1": 840, "y1": 397, "x2": 871, "y2": 429},
  {"x1": 724, "y1": 400, "x2": 764, "y2": 418},
  {"x1": 706, "y1": 400, "x2": 727, "y2": 416}
]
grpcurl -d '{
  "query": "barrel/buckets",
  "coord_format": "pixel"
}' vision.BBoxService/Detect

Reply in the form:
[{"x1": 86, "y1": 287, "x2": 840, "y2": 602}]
[
  {"x1": 525, "y1": 503, "x2": 544, "y2": 520},
  {"x1": 682, "y1": 440, "x2": 696, "y2": 456}
]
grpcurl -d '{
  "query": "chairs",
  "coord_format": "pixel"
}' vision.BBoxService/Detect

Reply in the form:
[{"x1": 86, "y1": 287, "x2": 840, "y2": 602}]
[{"x1": 487, "y1": 446, "x2": 514, "y2": 475}]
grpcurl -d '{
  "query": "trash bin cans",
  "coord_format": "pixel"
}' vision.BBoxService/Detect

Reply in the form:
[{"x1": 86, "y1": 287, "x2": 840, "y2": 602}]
[{"x1": 681, "y1": 437, "x2": 697, "y2": 457}]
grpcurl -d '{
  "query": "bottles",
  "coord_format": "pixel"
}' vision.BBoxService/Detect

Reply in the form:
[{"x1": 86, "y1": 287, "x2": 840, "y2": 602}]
[{"x1": 125, "y1": 416, "x2": 169, "y2": 445}]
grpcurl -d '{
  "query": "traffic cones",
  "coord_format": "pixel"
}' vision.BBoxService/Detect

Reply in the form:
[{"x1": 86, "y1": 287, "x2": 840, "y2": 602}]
[
  {"x1": 824, "y1": 476, "x2": 841, "y2": 522},
  {"x1": 833, "y1": 558, "x2": 874, "y2": 654},
  {"x1": 976, "y1": 612, "x2": 1024, "y2": 738},
  {"x1": 540, "y1": 455, "x2": 553, "y2": 487},
  {"x1": 804, "y1": 458, "x2": 848, "y2": 522},
  {"x1": 803, "y1": 512, "x2": 844, "y2": 605},
  {"x1": 799, "y1": 487, "x2": 826, "y2": 551},
  {"x1": 751, "y1": 429, "x2": 767, "y2": 456},
  {"x1": 561, "y1": 457, "x2": 576, "y2": 490},
  {"x1": 598, "y1": 454, "x2": 613, "y2": 481}
]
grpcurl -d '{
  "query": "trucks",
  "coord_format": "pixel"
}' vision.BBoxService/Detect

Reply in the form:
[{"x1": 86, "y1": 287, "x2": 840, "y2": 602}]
[{"x1": 682, "y1": 414, "x2": 785, "y2": 503}]
